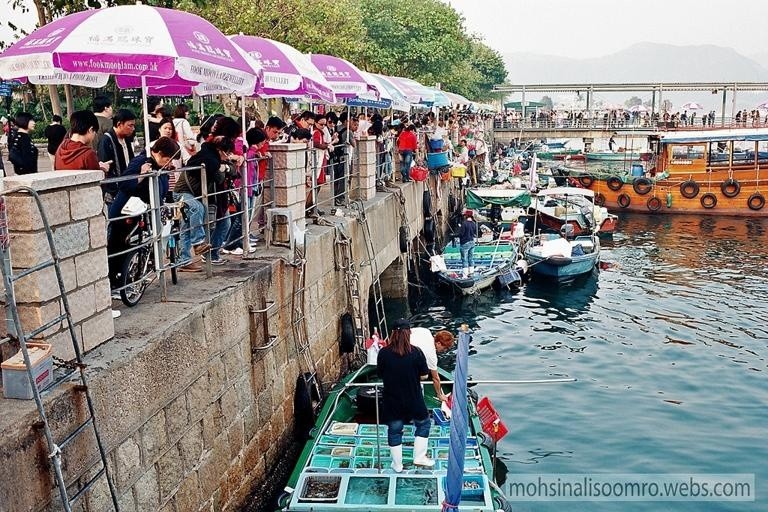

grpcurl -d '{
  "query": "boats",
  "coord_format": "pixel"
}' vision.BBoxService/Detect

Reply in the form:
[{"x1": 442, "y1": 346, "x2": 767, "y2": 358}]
[
  {"x1": 520, "y1": 146, "x2": 581, "y2": 158},
  {"x1": 525, "y1": 234, "x2": 600, "y2": 283},
  {"x1": 530, "y1": 188, "x2": 618, "y2": 233},
  {"x1": 558, "y1": 128, "x2": 768, "y2": 216},
  {"x1": 436, "y1": 233, "x2": 521, "y2": 294},
  {"x1": 527, "y1": 140, "x2": 569, "y2": 149},
  {"x1": 274, "y1": 365, "x2": 511, "y2": 512},
  {"x1": 584, "y1": 148, "x2": 651, "y2": 161},
  {"x1": 462, "y1": 188, "x2": 531, "y2": 231}
]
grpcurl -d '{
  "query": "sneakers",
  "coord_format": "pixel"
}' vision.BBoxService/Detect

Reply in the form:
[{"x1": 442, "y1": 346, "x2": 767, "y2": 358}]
[
  {"x1": 223, "y1": 247, "x2": 244, "y2": 255},
  {"x1": 202, "y1": 258, "x2": 225, "y2": 265},
  {"x1": 336, "y1": 197, "x2": 346, "y2": 206},
  {"x1": 217, "y1": 257, "x2": 228, "y2": 262},
  {"x1": 249, "y1": 247, "x2": 256, "y2": 252},
  {"x1": 249, "y1": 234, "x2": 259, "y2": 241},
  {"x1": 239, "y1": 239, "x2": 257, "y2": 246}
]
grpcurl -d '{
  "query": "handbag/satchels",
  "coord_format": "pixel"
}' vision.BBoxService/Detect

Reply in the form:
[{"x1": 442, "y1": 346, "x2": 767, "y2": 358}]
[
  {"x1": 307, "y1": 130, "x2": 325, "y2": 179},
  {"x1": 184, "y1": 171, "x2": 217, "y2": 230},
  {"x1": 230, "y1": 187, "x2": 241, "y2": 204},
  {"x1": 102, "y1": 133, "x2": 121, "y2": 220},
  {"x1": 182, "y1": 118, "x2": 196, "y2": 151}
]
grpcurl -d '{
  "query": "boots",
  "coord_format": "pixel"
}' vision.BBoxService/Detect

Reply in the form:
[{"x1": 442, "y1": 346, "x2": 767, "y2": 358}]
[
  {"x1": 389, "y1": 443, "x2": 403, "y2": 472},
  {"x1": 462, "y1": 268, "x2": 468, "y2": 279},
  {"x1": 469, "y1": 266, "x2": 474, "y2": 276},
  {"x1": 412, "y1": 436, "x2": 436, "y2": 466}
]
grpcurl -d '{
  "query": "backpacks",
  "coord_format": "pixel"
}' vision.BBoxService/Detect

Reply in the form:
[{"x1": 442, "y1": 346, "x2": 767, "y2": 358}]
[{"x1": 333, "y1": 126, "x2": 347, "y2": 168}]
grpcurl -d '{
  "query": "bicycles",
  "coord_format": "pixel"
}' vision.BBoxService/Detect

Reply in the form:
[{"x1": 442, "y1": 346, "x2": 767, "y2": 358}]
[{"x1": 120, "y1": 201, "x2": 189, "y2": 307}]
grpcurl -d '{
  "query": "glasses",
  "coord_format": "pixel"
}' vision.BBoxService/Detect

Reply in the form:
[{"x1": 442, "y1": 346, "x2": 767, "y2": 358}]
[
  {"x1": 222, "y1": 150, "x2": 232, "y2": 160},
  {"x1": 317, "y1": 123, "x2": 327, "y2": 126},
  {"x1": 327, "y1": 120, "x2": 336, "y2": 124},
  {"x1": 304, "y1": 119, "x2": 314, "y2": 126}
]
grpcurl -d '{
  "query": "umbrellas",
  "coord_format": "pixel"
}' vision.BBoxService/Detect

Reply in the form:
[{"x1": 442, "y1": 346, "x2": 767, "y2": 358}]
[
  {"x1": 756, "y1": 100, "x2": 768, "y2": 110},
  {"x1": 369, "y1": 73, "x2": 497, "y2": 113},
  {"x1": 0, "y1": 2, "x2": 265, "y2": 276},
  {"x1": 628, "y1": 105, "x2": 650, "y2": 112},
  {"x1": 146, "y1": 31, "x2": 336, "y2": 257},
  {"x1": 284, "y1": 71, "x2": 393, "y2": 198},
  {"x1": 303, "y1": 51, "x2": 380, "y2": 204},
  {"x1": 680, "y1": 102, "x2": 704, "y2": 110}
]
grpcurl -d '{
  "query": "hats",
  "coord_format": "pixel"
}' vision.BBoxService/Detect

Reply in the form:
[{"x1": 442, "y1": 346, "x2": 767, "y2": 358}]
[
  {"x1": 393, "y1": 317, "x2": 410, "y2": 330},
  {"x1": 464, "y1": 210, "x2": 473, "y2": 216}
]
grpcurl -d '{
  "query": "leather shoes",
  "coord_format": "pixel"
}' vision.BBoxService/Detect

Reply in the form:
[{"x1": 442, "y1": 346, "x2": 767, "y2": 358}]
[
  {"x1": 178, "y1": 263, "x2": 203, "y2": 272},
  {"x1": 194, "y1": 243, "x2": 212, "y2": 256}
]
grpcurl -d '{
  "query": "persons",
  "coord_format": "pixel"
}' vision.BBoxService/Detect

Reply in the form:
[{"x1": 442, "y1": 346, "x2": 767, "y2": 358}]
[
  {"x1": 288, "y1": 111, "x2": 315, "y2": 171},
  {"x1": 210, "y1": 117, "x2": 245, "y2": 265},
  {"x1": 396, "y1": 125, "x2": 417, "y2": 183},
  {"x1": 508, "y1": 139, "x2": 515, "y2": 157},
  {"x1": 735, "y1": 110, "x2": 768, "y2": 128},
  {"x1": 367, "y1": 114, "x2": 371, "y2": 121},
  {"x1": 89, "y1": 96, "x2": 113, "y2": 161},
  {"x1": 305, "y1": 114, "x2": 334, "y2": 218},
  {"x1": 45, "y1": 115, "x2": 69, "y2": 171},
  {"x1": 367, "y1": 114, "x2": 382, "y2": 130},
  {"x1": 172, "y1": 104, "x2": 197, "y2": 166},
  {"x1": 197, "y1": 116, "x2": 218, "y2": 143},
  {"x1": 8, "y1": 116, "x2": 14, "y2": 151},
  {"x1": 663, "y1": 111, "x2": 716, "y2": 128},
  {"x1": 394, "y1": 117, "x2": 408, "y2": 137},
  {"x1": 492, "y1": 155, "x2": 502, "y2": 177},
  {"x1": 223, "y1": 115, "x2": 258, "y2": 254},
  {"x1": 254, "y1": 119, "x2": 264, "y2": 128},
  {"x1": 245, "y1": 128, "x2": 265, "y2": 246},
  {"x1": 338, "y1": 112, "x2": 351, "y2": 131},
  {"x1": 368, "y1": 120, "x2": 384, "y2": 143},
  {"x1": 97, "y1": 108, "x2": 139, "y2": 211},
  {"x1": 407, "y1": 327, "x2": 454, "y2": 403},
  {"x1": 377, "y1": 318, "x2": 435, "y2": 472},
  {"x1": 142, "y1": 95, "x2": 159, "y2": 148},
  {"x1": 530, "y1": 108, "x2": 588, "y2": 128},
  {"x1": 53, "y1": 110, "x2": 121, "y2": 319},
  {"x1": 495, "y1": 113, "x2": 523, "y2": 128},
  {"x1": 644, "y1": 111, "x2": 659, "y2": 127},
  {"x1": 335, "y1": 118, "x2": 359, "y2": 206},
  {"x1": 145, "y1": 118, "x2": 199, "y2": 204},
  {"x1": 107, "y1": 137, "x2": 181, "y2": 300},
  {"x1": 380, "y1": 128, "x2": 386, "y2": 177},
  {"x1": 385, "y1": 125, "x2": 399, "y2": 181},
  {"x1": 281, "y1": 129, "x2": 311, "y2": 144},
  {"x1": 236, "y1": 116, "x2": 250, "y2": 135},
  {"x1": 631, "y1": 111, "x2": 641, "y2": 125},
  {"x1": 603, "y1": 109, "x2": 630, "y2": 128},
  {"x1": 511, "y1": 218, "x2": 518, "y2": 234},
  {"x1": 358, "y1": 114, "x2": 365, "y2": 121},
  {"x1": 215, "y1": 113, "x2": 224, "y2": 118},
  {"x1": 593, "y1": 112, "x2": 599, "y2": 127},
  {"x1": 449, "y1": 210, "x2": 476, "y2": 279},
  {"x1": 173, "y1": 136, "x2": 237, "y2": 273},
  {"x1": 250, "y1": 116, "x2": 286, "y2": 239},
  {"x1": 325, "y1": 111, "x2": 339, "y2": 175},
  {"x1": 147, "y1": 107, "x2": 179, "y2": 143},
  {"x1": 250, "y1": 116, "x2": 255, "y2": 129},
  {"x1": 410, "y1": 111, "x2": 494, "y2": 182},
  {"x1": 608, "y1": 132, "x2": 617, "y2": 150},
  {"x1": 10, "y1": 111, "x2": 38, "y2": 175}
]
